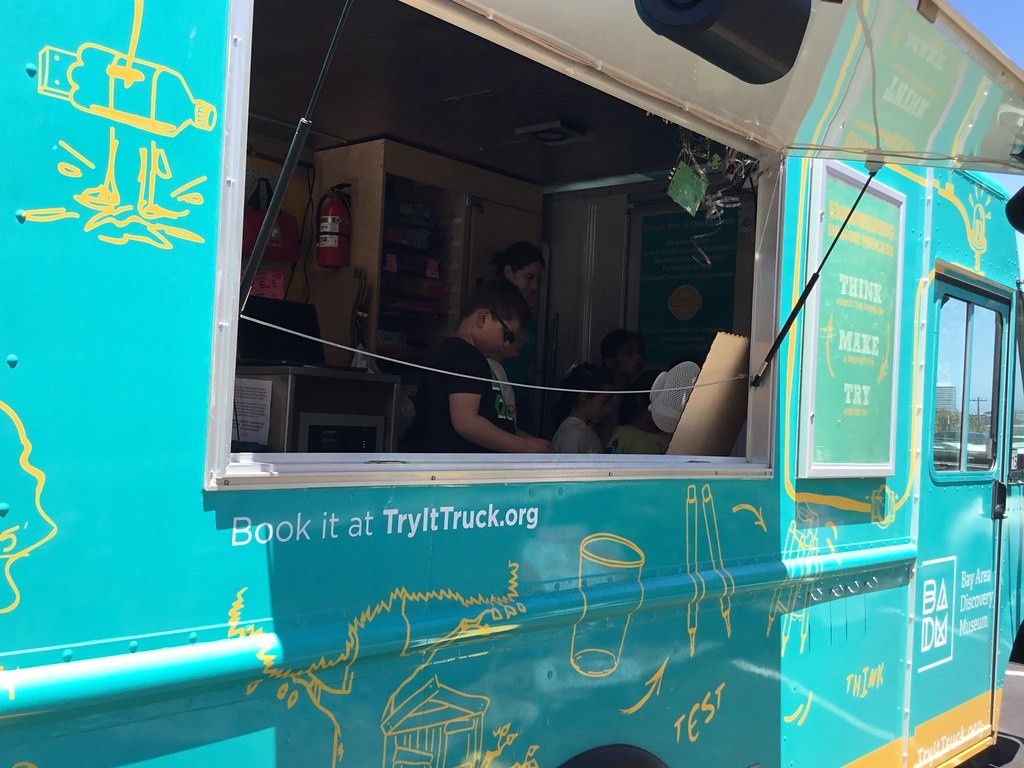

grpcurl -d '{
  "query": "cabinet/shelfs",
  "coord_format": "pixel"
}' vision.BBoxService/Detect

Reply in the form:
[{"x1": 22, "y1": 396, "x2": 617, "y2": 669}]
[{"x1": 307, "y1": 137, "x2": 545, "y2": 358}]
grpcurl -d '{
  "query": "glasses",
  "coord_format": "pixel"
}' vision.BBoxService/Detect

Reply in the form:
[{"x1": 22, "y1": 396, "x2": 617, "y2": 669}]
[{"x1": 489, "y1": 309, "x2": 516, "y2": 345}]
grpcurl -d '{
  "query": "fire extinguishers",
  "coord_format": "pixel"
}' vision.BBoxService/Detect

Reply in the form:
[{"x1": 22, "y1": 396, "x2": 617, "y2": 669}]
[{"x1": 316, "y1": 182, "x2": 352, "y2": 270}]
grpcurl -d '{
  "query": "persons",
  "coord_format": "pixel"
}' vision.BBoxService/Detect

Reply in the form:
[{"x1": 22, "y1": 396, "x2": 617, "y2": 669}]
[{"x1": 424, "y1": 240, "x2": 703, "y2": 454}]
